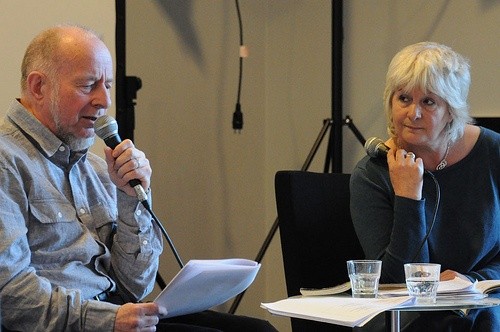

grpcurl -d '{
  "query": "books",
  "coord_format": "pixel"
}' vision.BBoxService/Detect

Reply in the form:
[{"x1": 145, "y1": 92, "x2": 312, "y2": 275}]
[{"x1": 300, "y1": 276, "x2": 500, "y2": 300}]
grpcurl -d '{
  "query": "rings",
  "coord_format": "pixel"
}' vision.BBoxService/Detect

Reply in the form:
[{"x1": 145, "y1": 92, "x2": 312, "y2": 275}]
[
  {"x1": 407, "y1": 153, "x2": 415, "y2": 159},
  {"x1": 135, "y1": 158, "x2": 140, "y2": 169}
]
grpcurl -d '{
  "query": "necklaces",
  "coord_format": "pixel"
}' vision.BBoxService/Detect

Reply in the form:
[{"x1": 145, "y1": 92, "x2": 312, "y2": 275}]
[{"x1": 435, "y1": 142, "x2": 450, "y2": 170}]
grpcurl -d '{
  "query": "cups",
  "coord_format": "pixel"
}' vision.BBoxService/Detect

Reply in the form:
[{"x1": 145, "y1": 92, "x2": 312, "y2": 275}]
[
  {"x1": 403, "y1": 263, "x2": 441, "y2": 305},
  {"x1": 347, "y1": 260, "x2": 382, "y2": 298}
]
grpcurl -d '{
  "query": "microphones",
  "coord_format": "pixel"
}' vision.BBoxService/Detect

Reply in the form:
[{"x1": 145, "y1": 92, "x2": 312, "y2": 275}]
[
  {"x1": 93, "y1": 115, "x2": 150, "y2": 211},
  {"x1": 365, "y1": 137, "x2": 435, "y2": 178}
]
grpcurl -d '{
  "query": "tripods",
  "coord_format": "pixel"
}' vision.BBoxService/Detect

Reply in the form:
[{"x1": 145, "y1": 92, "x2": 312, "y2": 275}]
[{"x1": 228, "y1": 0, "x2": 366, "y2": 315}]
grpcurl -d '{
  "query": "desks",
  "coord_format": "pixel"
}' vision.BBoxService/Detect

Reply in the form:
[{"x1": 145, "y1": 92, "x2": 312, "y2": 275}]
[{"x1": 385, "y1": 296, "x2": 500, "y2": 332}]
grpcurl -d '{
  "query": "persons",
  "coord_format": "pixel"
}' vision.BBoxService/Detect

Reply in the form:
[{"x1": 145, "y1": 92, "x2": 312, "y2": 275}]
[
  {"x1": 0, "y1": 24, "x2": 277, "y2": 332},
  {"x1": 350, "y1": 41, "x2": 500, "y2": 332}
]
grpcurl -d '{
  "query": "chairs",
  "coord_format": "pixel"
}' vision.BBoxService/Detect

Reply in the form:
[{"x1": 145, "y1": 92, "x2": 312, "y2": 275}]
[{"x1": 275, "y1": 170, "x2": 421, "y2": 332}]
